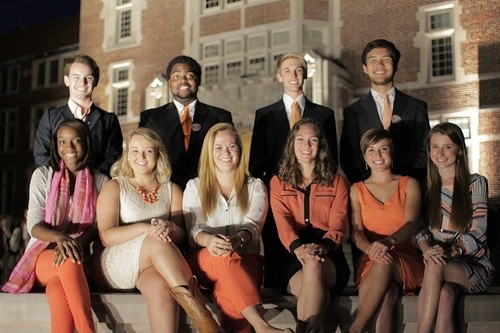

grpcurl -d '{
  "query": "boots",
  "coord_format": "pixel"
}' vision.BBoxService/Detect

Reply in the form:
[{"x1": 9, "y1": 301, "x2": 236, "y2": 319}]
[
  {"x1": 169, "y1": 275, "x2": 225, "y2": 333},
  {"x1": 296, "y1": 315, "x2": 323, "y2": 333}
]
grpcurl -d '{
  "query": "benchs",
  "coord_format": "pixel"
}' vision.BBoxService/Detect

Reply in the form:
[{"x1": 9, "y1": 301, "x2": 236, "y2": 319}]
[{"x1": 0, "y1": 292, "x2": 500, "y2": 333}]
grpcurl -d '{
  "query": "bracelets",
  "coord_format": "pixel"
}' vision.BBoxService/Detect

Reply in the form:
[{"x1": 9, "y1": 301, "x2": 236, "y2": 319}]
[
  {"x1": 171, "y1": 222, "x2": 175, "y2": 235},
  {"x1": 236, "y1": 233, "x2": 245, "y2": 249}
]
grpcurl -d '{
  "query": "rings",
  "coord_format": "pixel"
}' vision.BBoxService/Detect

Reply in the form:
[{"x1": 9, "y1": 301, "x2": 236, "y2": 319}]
[{"x1": 215, "y1": 241, "x2": 219, "y2": 247}]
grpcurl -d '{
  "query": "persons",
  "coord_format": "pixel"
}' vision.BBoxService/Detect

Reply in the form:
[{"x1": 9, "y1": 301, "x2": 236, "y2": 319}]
[
  {"x1": 182, "y1": 122, "x2": 294, "y2": 333},
  {"x1": 34, "y1": 55, "x2": 123, "y2": 294},
  {"x1": 0, "y1": 216, "x2": 24, "y2": 293},
  {"x1": 97, "y1": 127, "x2": 224, "y2": 333},
  {"x1": 271, "y1": 117, "x2": 349, "y2": 333},
  {"x1": 349, "y1": 128, "x2": 425, "y2": 333},
  {"x1": 137, "y1": 56, "x2": 235, "y2": 260},
  {"x1": 408, "y1": 122, "x2": 489, "y2": 333},
  {"x1": 340, "y1": 39, "x2": 432, "y2": 286},
  {"x1": 247, "y1": 53, "x2": 339, "y2": 288},
  {"x1": 25, "y1": 117, "x2": 110, "y2": 333}
]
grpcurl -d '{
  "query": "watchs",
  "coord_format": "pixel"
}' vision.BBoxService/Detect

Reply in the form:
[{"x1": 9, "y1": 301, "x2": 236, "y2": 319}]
[{"x1": 453, "y1": 243, "x2": 463, "y2": 256}]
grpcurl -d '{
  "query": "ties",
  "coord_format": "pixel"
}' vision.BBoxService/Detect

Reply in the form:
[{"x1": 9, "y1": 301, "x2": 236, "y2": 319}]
[
  {"x1": 181, "y1": 107, "x2": 192, "y2": 151},
  {"x1": 289, "y1": 101, "x2": 302, "y2": 131},
  {"x1": 379, "y1": 93, "x2": 392, "y2": 130}
]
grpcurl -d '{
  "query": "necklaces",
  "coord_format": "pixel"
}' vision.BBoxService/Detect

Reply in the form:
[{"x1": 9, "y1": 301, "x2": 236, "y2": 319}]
[
  {"x1": 222, "y1": 191, "x2": 231, "y2": 198},
  {"x1": 132, "y1": 175, "x2": 161, "y2": 205}
]
